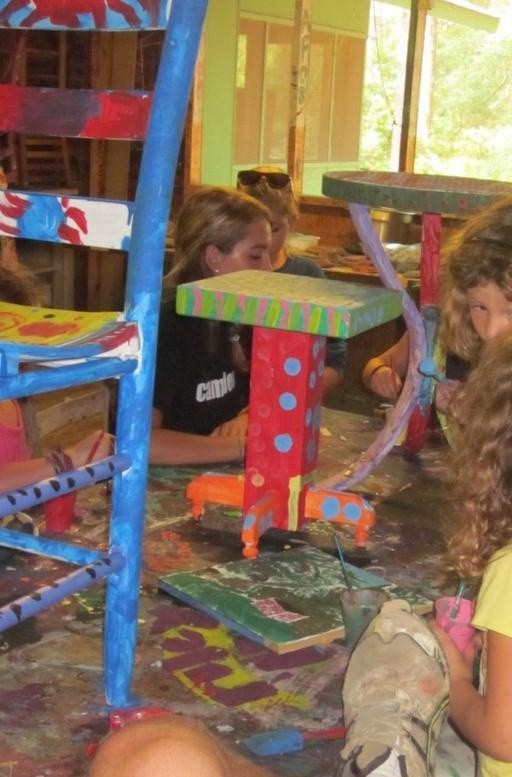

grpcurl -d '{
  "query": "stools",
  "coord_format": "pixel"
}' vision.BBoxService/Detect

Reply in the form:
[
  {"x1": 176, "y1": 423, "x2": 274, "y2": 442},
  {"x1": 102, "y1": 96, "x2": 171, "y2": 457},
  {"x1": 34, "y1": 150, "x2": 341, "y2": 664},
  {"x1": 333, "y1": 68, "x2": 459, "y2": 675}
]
[
  {"x1": 322, "y1": 171, "x2": 512, "y2": 461},
  {"x1": 174, "y1": 267, "x2": 405, "y2": 559}
]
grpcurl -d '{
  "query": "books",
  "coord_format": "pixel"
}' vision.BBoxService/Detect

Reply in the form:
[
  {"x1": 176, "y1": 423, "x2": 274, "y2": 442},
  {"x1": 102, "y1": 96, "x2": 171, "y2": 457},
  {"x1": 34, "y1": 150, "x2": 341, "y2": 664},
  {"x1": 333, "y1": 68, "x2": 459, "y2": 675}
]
[
  {"x1": 0, "y1": 300, "x2": 123, "y2": 349},
  {"x1": 157, "y1": 542, "x2": 436, "y2": 657}
]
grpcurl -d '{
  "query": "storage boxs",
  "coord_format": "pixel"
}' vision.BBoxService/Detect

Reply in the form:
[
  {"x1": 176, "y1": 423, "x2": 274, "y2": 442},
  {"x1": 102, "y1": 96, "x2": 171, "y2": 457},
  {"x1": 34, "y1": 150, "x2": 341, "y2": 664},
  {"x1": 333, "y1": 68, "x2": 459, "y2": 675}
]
[{"x1": 21, "y1": 382, "x2": 109, "y2": 459}]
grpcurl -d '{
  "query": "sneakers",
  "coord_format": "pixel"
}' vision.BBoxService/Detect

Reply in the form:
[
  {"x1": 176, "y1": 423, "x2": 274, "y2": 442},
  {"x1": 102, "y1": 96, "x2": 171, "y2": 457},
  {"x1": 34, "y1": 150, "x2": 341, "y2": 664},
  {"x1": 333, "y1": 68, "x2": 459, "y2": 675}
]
[{"x1": 338, "y1": 597, "x2": 452, "y2": 776}]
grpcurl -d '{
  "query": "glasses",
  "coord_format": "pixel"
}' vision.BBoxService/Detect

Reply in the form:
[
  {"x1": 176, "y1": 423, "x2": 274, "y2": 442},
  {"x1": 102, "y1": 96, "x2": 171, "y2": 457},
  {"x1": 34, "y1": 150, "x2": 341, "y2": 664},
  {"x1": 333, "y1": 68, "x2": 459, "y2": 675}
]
[{"x1": 237, "y1": 167, "x2": 292, "y2": 192}]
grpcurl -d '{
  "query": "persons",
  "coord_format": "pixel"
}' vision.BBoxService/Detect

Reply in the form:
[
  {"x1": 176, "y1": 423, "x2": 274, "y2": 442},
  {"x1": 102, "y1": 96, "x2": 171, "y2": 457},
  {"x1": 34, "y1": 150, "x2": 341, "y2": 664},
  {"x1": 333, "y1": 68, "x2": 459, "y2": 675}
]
[
  {"x1": 236, "y1": 165, "x2": 349, "y2": 398},
  {"x1": 361, "y1": 328, "x2": 412, "y2": 404},
  {"x1": 428, "y1": 327, "x2": 512, "y2": 776},
  {"x1": 434, "y1": 195, "x2": 512, "y2": 417},
  {"x1": 87, "y1": 597, "x2": 452, "y2": 776},
  {"x1": 148, "y1": 185, "x2": 275, "y2": 466}
]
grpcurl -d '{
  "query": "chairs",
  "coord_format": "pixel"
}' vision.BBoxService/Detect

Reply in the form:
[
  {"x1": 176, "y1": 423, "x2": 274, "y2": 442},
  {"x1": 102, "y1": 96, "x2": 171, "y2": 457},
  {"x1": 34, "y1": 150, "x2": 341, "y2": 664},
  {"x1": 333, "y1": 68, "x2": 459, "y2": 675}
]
[{"x1": 0, "y1": 0, "x2": 208, "y2": 713}]
